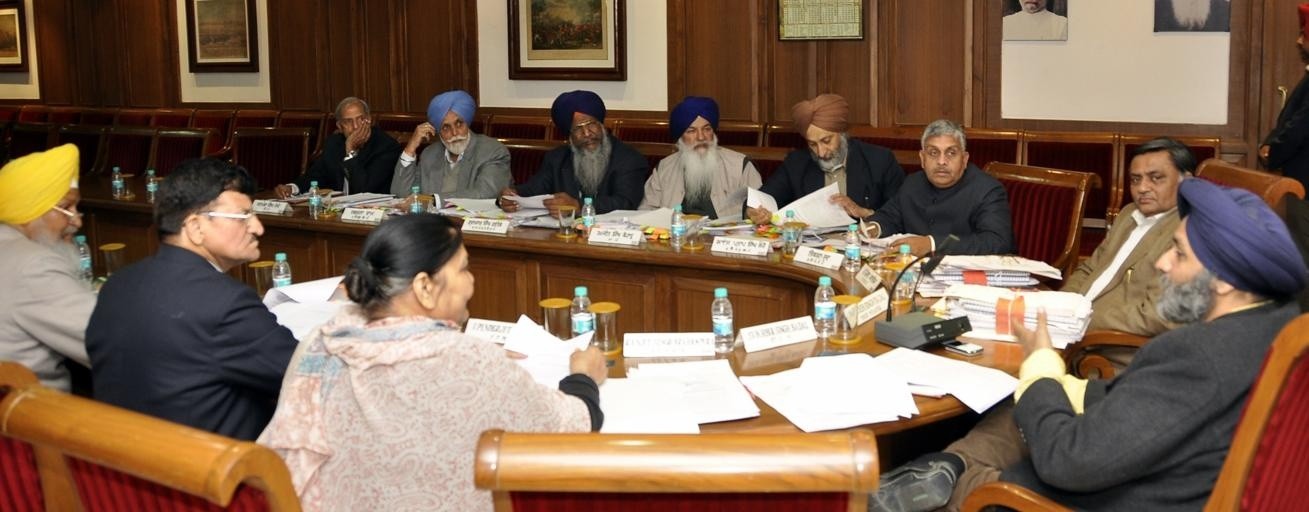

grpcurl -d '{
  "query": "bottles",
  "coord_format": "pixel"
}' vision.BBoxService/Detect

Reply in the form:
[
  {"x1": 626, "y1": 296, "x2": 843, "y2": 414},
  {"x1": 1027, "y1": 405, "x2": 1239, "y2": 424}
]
[
  {"x1": 304, "y1": 178, "x2": 326, "y2": 222},
  {"x1": 98, "y1": 242, "x2": 129, "y2": 282},
  {"x1": 69, "y1": 233, "x2": 94, "y2": 282},
  {"x1": 868, "y1": 244, "x2": 915, "y2": 321},
  {"x1": 567, "y1": 280, "x2": 599, "y2": 357},
  {"x1": 122, "y1": 173, "x2": 138, "y2": 202},
  {"x1": 808, "y1": 276, "x2": 838, "y2": 347},
  {"x1": 781, "y1": 210, "x2": 798, "y2": 257},
  {"x1": 588, "y1": 301, "x2": 625, "y2": 358},
  {"x1": 419, "y1": 194, "x2": 435, "y2": 216},
  {"x1": 581, "y1": 196, "x2": 597, "y2": 240},
  {"x1": 784, "y1": 221, "x2": 809, "y2": 259},
  {"x1": 246, "y1": 258, "x2": 279, "y2": 302},
  {"x1": 829, "y1": 293, "x2": 863, "y2": 344},
  {"x1": 272, "y1": 252, "x2": 293, "y2": 289},
  {"x1": 842, "y1": 222, "x2": 867, "y2": 273},
  {"x1": 110, "y1": 166, "x2": 127, "y2": 201},
  {"x1": 407, "y1": 185, "x2": 425, "y2": 217},
  {"x1": 669, "y1": 204, "x2": 686, "y2": 252},
  {"x1": 557, "y1": 205, "x2": 578, "y2": 240},
  {"x1": 711, "y1": 284, "x2": 737, "y2": 359},
  {"x1": 146, "y1": 169, "x2": 160, "y2": 203},
  {"x1": 679, "y1": 213, "x2": 708, "y2": 251},
  {"x1": 537, "y1": 297, "x2": 574, "y2": 352}
]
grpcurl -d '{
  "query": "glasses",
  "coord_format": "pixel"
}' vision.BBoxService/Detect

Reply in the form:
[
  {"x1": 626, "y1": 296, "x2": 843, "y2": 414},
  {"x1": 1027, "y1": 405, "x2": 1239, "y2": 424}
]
[
  {"x1": 49, "y1": 202, "x2": 84, "y2": 227},
  {"x1": 195, "y1": 207, "x2": 257, "y2": 223}
]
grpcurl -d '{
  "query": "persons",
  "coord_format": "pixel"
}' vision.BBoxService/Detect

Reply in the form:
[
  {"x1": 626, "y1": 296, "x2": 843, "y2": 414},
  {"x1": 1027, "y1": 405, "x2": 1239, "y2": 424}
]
[
  {"x1": 1256, "y1": 0, "x2": 1309, "y2": 284},
  {"x1": 867, "y1": 174, "x2": 1308, "y2": 512},
  {"x1": 255, "y1": 211, "x2": 609, "y2": 512},
  {"x1": 82, "y1": 157, "x2": 300, "y2": 442},
  {"x1": 1002, "y1": 0, "x2": 1068, "y2": 41},
  {"x1": 0, "y1": 143, "x2": 101, "y2": 397},
  {"x1": 1055, "y1": 136, "x2": 1199, "y2": 378}
]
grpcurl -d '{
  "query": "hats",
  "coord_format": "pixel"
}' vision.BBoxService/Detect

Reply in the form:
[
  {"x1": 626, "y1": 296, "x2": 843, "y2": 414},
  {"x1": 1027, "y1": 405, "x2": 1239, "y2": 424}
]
[
  {"x1": 791, "y1": 92, "x2": 850, "y2": 137},
  {"x1": 550, "y1": 89, "x2": 606, "y2": 134},
  {"x1": 1175, "y1": 175, "x2": 1307, "y2": 303},
  {"x1": 668, "y1": 95, "x2": 720, "y2": 136},
  {"x1": 0, "y1": 144, "x2": 82, "y2": 226},
  {"x1": 426, "y1": 90, "x2": 477, "y2": 133}
]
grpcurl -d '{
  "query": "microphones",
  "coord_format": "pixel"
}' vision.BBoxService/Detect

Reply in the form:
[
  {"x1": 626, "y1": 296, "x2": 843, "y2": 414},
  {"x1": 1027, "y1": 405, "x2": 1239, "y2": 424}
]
[{"x1": 874, "y1": 233, "x2": 972, "y2": 350}]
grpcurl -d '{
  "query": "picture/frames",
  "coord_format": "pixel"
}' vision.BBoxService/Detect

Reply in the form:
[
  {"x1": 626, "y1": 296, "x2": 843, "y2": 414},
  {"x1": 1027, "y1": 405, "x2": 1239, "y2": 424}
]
[
  {"x1": 0, "y1": 0, "x2": 30, "y2": 73},
  {"x1": 185, "y1": 0, "x2": 260, "y2": 73},
  {"x1": 506, "y1": 0, "x2": 627, "y2": 82}
]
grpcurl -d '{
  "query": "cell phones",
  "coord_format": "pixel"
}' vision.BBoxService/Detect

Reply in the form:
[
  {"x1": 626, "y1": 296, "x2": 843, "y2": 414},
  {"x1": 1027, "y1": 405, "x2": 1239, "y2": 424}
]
[{"x1": 943, "y1": 338, "x2": 985, "y2": 357}]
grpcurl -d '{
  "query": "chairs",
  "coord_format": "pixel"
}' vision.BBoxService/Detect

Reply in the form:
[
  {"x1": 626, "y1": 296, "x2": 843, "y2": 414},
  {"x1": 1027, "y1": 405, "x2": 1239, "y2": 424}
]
[
  {"x1": 0, "y1": 385, "x2": 304, "y2": 512},
  {"x1": 0, "y1": 360, "x2": 82, "y2": 511},
  {"x1": 1068, "y1": 158, "x2": 1308, "y2": 382},
  {"x1": 957, "y1": 315, "x2": 1306, "y2": 511},
  {"x1": 471, "y1": 426, "x2": 881, "y2": 512},
  {"x1": 0, "y1": 101, "x2": 1221, "y2": 433}
]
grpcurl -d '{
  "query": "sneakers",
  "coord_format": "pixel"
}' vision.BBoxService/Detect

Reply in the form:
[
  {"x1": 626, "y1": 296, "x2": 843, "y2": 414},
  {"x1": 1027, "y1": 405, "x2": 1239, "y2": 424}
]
[{"x1": 865, "y1": 461, "x2": 961, "y2": 512}]
